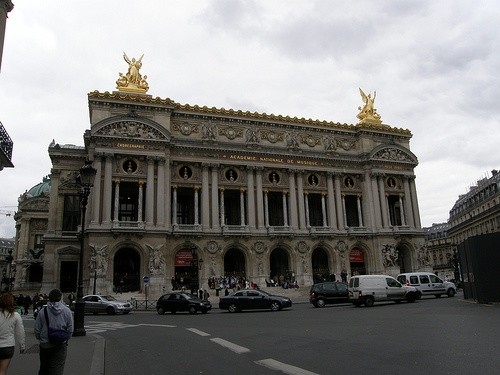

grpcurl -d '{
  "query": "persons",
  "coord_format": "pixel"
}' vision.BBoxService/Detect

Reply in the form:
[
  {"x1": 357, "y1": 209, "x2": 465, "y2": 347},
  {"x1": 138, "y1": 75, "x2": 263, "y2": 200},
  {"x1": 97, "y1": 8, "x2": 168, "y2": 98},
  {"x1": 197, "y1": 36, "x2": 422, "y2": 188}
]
[
  {"x1": 0, "y1": 294, "x2": 25, "y2": 375},
  {"x1": 266, "y1": 272, "x2": 295, "y2": 289},
  {"x1": 341, "y1": 269, "x2": 347, "y2": 283},
  {"x1": 34, "y1": 288, "x2": 73, "y2": 375},
  {"x1": 225, "y1": 288, "x2": 229, "y2": 296},
  {"x1": 385, "y1": 246, "x2": 399, "y2": 266},
  {"x1": 320, "y1": 272, "x2": 335, "y2": 281},
  {"x1": 0, "y1": 291, "x2": 48, "y2": 315},
  {"x1": 208, "y1": 276, "x2": 260, "y2": 290},
  {"x1": 171, "y1": 276, "x2": 208, "y2": 301},
  {"x1": 116, "y1": 51, "x2": 149, "y2": 90},
  {"x1": 356, "y1": 87, "x2": 376, "y2": 118},
  {"x1": 68, "y1": 294, "x2": 72, "y2": 307}
]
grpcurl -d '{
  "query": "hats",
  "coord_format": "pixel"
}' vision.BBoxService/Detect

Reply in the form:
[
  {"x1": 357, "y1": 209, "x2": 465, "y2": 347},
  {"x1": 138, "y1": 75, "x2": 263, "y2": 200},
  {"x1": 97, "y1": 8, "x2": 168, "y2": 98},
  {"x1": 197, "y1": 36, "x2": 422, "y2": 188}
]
[{"x1": 49, "y1": 288, "x2": 62, "y2": 295}]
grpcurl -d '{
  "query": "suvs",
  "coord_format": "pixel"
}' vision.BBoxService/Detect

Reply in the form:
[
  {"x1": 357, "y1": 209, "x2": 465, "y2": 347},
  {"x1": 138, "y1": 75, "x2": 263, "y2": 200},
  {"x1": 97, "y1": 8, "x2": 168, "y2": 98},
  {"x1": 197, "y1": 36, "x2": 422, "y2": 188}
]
[
  {"x1": 348, "y1": 275, "x2": 421, "y2": 306},
  {"x1": 310, "y1": 281, "x2": 361, "y2": 308},
  {"x1": 396, "y1": 272, "x2": 456, "y2": 300}
]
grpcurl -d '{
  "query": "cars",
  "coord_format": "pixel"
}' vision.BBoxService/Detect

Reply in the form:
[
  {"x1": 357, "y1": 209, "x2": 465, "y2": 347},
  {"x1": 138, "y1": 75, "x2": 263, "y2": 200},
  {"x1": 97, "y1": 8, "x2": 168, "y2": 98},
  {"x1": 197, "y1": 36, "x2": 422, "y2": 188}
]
[
  {"x1": 219, "y1": 289, "x2": 292, "y2": 313},
  {"x1": 70, "y1": 294, "x2": 132, "y2": 315},
  {"x1": 156, "y1": 291, "x2": 212, "y2": 315}
]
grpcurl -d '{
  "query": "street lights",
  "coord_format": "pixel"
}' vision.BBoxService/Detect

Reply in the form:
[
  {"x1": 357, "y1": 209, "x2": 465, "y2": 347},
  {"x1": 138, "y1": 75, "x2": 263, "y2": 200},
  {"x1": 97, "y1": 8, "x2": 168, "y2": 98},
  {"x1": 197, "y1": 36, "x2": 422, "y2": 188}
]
[
  {"x1": 72, "y1": 162, "x2": 97, "y2": 336},
  {"x1": 7, "y1": 249, "x2": 13, "y2": 291}
]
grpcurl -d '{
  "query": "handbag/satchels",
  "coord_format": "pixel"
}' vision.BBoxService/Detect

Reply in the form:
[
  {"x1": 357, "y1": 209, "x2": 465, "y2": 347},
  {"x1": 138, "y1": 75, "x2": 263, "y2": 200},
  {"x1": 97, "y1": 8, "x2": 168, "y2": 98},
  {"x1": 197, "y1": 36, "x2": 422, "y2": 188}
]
[{"x1": 47, "y1": 327, "x2": 69, "y2": 345}]
